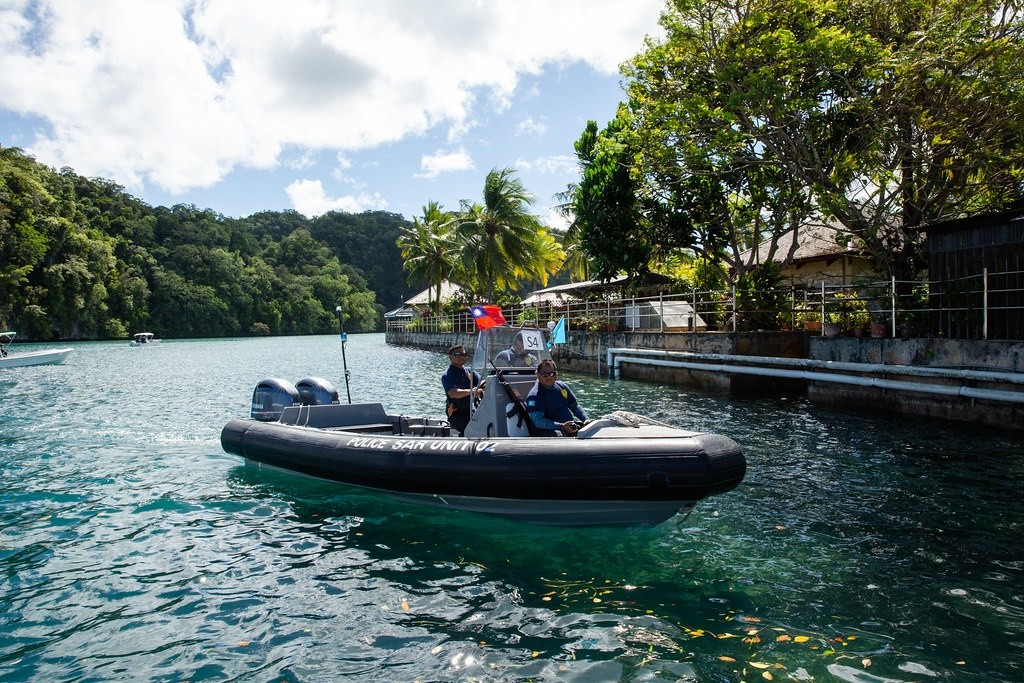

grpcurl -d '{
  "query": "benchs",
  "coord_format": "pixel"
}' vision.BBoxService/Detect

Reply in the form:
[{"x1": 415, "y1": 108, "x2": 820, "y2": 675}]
[{"x1": 280, "y1": 403, "x2": 393, "y2": 434}]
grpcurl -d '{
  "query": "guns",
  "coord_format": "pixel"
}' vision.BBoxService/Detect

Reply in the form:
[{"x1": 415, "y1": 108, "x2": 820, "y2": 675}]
[{"x1": 487, "y1": 358, "x2": 535, "y2": 436}]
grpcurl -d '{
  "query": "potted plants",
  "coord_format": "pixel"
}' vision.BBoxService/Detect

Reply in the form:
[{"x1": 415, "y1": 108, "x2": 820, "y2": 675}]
[
  {"x1": 517, "y1": 309, "x2": 622, "y2": 331},
  {"x1": 867, "y1": 313, "x2": 888, "y2": 339},
  {"x1": 822, "y1": 302, "x2": 842, "y2": 339},
  {"x1": 896, "y1": 312, "x2": 915, "y2": 337},
  {"x1": 850, "y1": 313, "x2": 868, "y2": 336},
  {"x1": 795, "y1": 302, "x2": 821, "y2": 331}
]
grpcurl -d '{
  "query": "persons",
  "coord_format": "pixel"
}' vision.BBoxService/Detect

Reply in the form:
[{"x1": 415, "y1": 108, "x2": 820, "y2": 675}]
[
  {"x1": 526, "y1": 358, "x2": 596, "y2": 438},
  {"x1": 493, "y1": 332, "x2": 538, "y2": 368},
  {"x1": 441, "y1": 344, "x2": 485, "y2": 437}
]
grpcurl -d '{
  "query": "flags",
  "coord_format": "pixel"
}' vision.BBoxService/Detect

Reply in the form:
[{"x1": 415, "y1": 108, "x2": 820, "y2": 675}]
[
  {"x1": 546, "y1": 315, "x2": 566, "y2": 348},
  {"x1": 470, "y1": 305, "x2": 507, "y2": 330}
]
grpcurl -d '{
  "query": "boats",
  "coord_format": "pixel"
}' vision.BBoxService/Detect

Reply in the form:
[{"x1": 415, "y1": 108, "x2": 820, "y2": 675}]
[
  {"x1": 218, "y1": 306, "x2": 748, "y2": 529},
  {"x1": 0, "y1": 332, "x2": 75, "y2": 368},
  {"x1": 128, "y1": 332, "x2": 162, "y2": 347}
]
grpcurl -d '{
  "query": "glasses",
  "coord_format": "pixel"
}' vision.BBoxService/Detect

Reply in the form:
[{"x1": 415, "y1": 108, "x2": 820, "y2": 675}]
[
  {"x1": 537, "y1": 371, "x2": 557, "y2": 378},
  {"x1": 449, "y1": 352, "x2": 467, "y2": 358}
]
